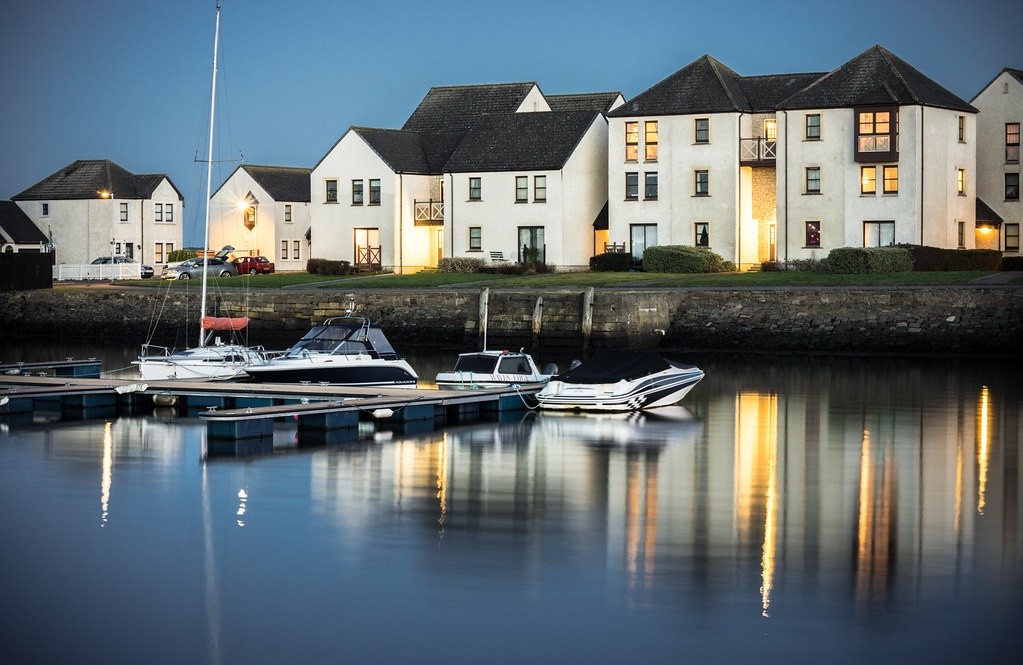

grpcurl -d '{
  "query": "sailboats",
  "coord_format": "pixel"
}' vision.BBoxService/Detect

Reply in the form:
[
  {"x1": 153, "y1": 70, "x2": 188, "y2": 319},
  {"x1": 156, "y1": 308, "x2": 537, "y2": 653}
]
[{"x1": 130, "y1": 1, "x2": 268, "y2": 382}]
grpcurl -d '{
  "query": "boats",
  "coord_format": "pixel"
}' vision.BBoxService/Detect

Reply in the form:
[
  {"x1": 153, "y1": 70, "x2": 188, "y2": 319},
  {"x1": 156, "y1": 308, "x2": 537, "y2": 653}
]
[
  {"x1": 241, "y1": 295, "x2": 420, "y2": 389},
  {"x1": 433, "y1": 291, "x2": 552, "y2": 391},
  {"x1": 535, "y1": 356, "x2": 706, "y2": 411}
]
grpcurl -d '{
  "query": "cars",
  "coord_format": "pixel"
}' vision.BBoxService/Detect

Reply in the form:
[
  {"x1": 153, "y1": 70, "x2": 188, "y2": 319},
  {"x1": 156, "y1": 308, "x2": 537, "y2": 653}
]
[
  {"x1": 230, "y1": 255, "x2": 274, "y2": 276},
  {"x1": 91, "y1": 256, "x2": 153, "y2": 278},
  {"x1": 160, "y1": 257, "x2": 239, "y2": 281}
]
